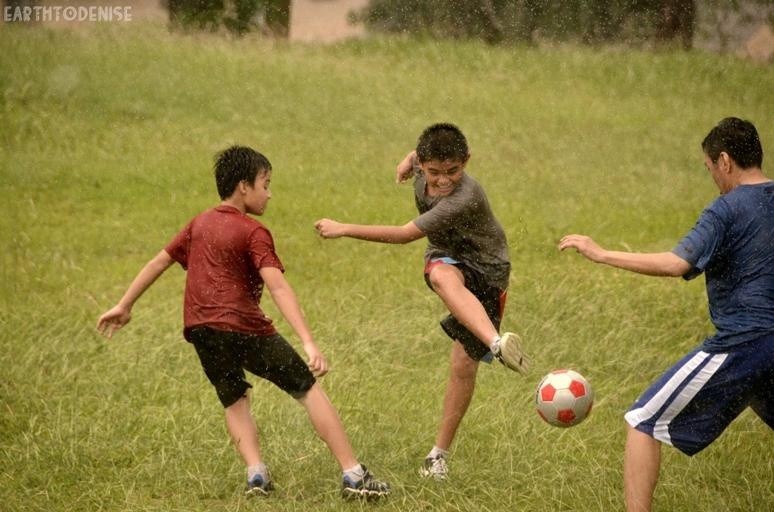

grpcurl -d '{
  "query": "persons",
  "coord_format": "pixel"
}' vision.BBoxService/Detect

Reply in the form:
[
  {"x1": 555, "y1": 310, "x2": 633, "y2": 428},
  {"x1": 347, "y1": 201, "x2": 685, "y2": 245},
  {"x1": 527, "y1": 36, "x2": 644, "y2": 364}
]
[
  {"x1": 313, "y1": 121, "x2": 531, "y2": 482},
  {"x1": 97, "y1": 143, "x2": 393, "y2": 503},
  {"x1": 559, "y1": 113, "x2": 774, "y2": 511}
]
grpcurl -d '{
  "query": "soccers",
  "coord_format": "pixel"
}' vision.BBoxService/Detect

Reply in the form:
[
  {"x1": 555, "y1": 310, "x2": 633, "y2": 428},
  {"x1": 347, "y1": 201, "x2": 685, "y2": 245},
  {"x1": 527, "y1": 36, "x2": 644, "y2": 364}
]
[{"x1": 536, "y1": 369, "x2": 592, "y2": 428}]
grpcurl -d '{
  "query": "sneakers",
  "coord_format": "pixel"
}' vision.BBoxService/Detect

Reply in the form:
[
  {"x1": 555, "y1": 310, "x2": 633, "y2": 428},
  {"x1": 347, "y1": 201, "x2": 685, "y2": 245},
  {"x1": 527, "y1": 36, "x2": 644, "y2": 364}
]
[
  {"x1": 341, "y1": 463, "x2": 391, "y2": 499},
  {"x1": 244, "y1": 472, "x2": 276, "y2": 497},
  {"x1": 490, "y1": 331, "x2": 534, "y2": 377},
  {"x1": 418, "y1": 452, "x2": 450, "y2": 480}
]
